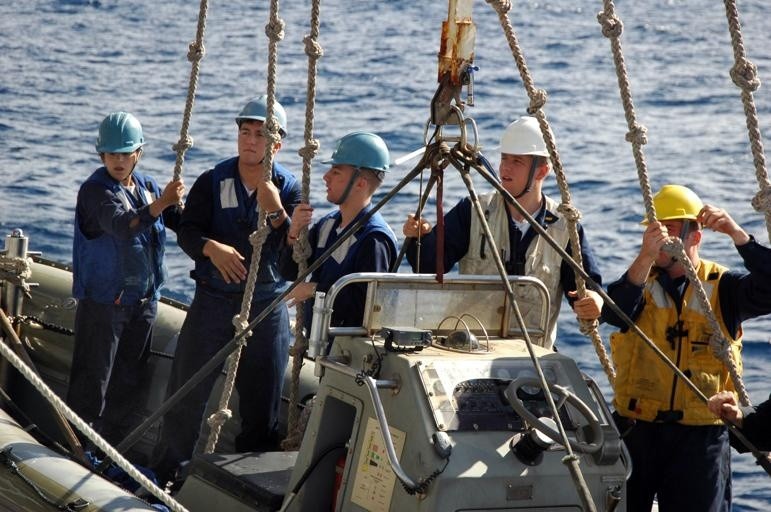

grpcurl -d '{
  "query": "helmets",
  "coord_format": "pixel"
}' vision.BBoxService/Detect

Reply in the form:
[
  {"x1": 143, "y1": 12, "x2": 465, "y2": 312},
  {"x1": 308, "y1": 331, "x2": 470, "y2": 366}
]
[
  {"x1": 320, "y1": 130, "x2": 392, "y2": 205},
  {"x1": 94, "y1": 110, "x2": 148, "y2": 181},
  {"x1": 235, "y1": 92, "x2": 289, "y2": 165},
  {"x1": 640, "y1": 184, "x2": 706, "y2": 273},
  {"x1": 500, "y1": 114, "x2": 549, "y2": 198}
]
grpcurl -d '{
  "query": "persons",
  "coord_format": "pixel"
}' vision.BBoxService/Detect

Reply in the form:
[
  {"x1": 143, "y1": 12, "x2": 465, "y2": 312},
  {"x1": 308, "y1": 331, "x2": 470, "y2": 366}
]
[
  {"x1": 606, "y1": 184, "x2": 771, "y2": 512},
  {"x1": 65, "y1": 110, "x2": 185, "y2": 466},
  {"x1": 145, "y1": 92, "x2": 302, "y2": 508},
  {"x1": 706, "y1": 391, "x2": 771, "y2": 455},
  {"x1": 276, "y1": 132, "x2": 398, "y2": 382},
  {"x1": 403, "y1": 117, "x2": 605, "y2": 353}
]
